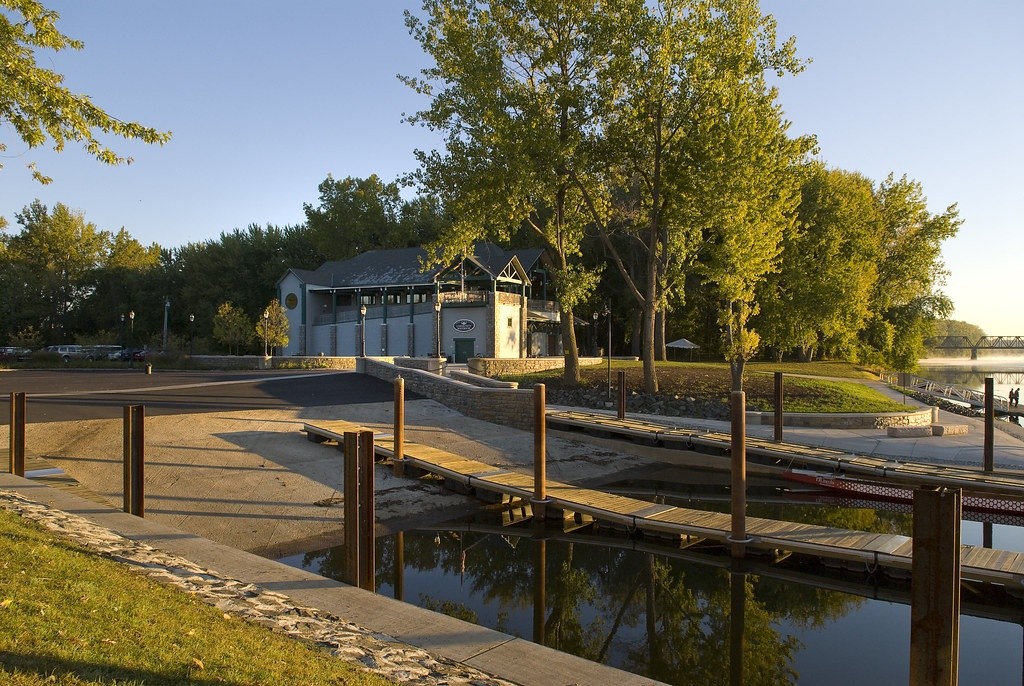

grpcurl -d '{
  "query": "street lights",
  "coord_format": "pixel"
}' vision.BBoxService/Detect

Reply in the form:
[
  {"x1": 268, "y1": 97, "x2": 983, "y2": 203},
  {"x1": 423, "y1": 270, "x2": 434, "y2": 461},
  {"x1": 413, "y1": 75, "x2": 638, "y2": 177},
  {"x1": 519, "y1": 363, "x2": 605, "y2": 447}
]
[
  {"x1": 601, "y1": 295, "x2": 613, "y2": 400},
  {"x1": 434, "y1": 300, "x2": 443, "y2": 358},
  {"x1": 593, "y1": 310, "x2": 599, "y2": 358},
  {"x1": 264, "y1": 310, "x2": 271, "y2": 356},
  {"x1": 360, "y1": 304, "x2": 367, "y2": 357},
  {"x1": 129, "y1": 310, "x2": 135, "y2": 331},
  {"x1": 163, "y1": 299, "x2": 171, "y2": 354},
  {"x1": 121, "y1": 314, "x2": 126, "y2": 362},
  {"x1": 190, "y1": 312, "x2": 196, "y2": 353}
]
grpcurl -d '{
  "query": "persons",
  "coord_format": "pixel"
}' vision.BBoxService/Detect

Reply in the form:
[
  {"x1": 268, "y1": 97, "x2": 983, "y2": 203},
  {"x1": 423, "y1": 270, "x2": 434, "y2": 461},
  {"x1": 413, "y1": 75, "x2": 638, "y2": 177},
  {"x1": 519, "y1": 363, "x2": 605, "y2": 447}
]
[
  {"x1": 1015, "y1": 388, "x2": 1020, "y2": 407},
  {"x1": 1009, "y1": 416, "x2": 1022, "y2": 427},
  {"x1": 1009, "y1": 388, "x2": 1014, "y2": 406}
]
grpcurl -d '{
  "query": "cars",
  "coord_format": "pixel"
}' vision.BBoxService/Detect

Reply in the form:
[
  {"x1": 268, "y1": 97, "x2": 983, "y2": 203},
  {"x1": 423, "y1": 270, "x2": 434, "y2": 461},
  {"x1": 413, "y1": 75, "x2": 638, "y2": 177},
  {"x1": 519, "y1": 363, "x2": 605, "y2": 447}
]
[{"x1": 0, "y1": 345, "x2": 147, "y2": 365}]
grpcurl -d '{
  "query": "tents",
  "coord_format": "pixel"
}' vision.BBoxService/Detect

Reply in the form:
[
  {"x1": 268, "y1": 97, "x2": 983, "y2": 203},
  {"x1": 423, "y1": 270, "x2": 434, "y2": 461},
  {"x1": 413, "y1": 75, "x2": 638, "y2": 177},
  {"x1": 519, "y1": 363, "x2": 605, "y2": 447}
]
[{"x1": 666, "y1": 339, "x2": 700, "y2": 362}]
